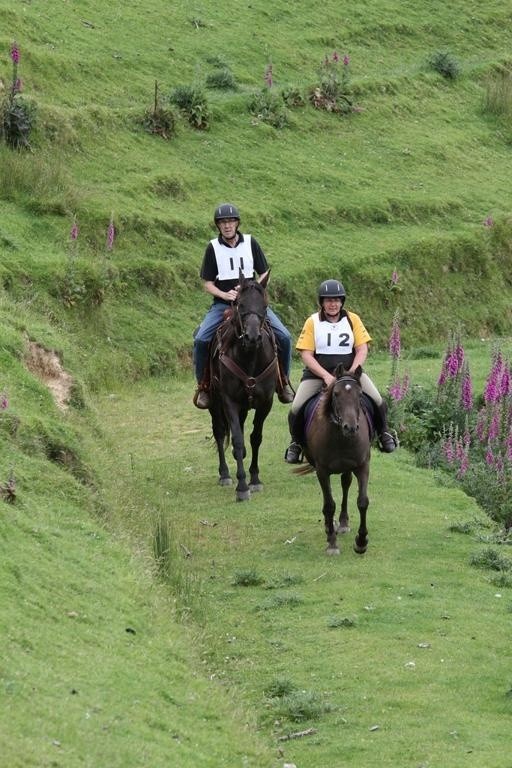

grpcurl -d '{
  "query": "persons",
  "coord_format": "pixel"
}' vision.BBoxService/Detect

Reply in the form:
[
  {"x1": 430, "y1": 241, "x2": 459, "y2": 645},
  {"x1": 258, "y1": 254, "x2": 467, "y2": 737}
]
[
  {"x1": 194, "y1": 205, "x2": 293, "y2": 409},
  {"x1": 286, "y1": 278, "x2": 396, "y2": 464}
]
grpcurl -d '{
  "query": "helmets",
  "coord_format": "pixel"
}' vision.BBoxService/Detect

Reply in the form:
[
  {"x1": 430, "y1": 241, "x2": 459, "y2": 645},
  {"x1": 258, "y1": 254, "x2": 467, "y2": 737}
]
[
  {"x1": 318, "y1": 279, "x2": 346, "y2": 303},
  {"x1": 215, "y1": 204, "x2": 240, "y2": 224}
]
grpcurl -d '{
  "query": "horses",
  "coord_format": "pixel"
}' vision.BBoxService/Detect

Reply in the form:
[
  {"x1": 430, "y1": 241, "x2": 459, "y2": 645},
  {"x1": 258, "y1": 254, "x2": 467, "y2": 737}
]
[
  {"x1": 290, "y1": 362, "x2": 377, "y2": 558},
  {"x1": 206, "y1": 265, "x2": 278, "y2": 503}
]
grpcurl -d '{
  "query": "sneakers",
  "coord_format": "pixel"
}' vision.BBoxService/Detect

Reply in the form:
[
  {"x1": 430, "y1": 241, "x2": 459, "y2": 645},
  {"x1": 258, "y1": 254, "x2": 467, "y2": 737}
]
[
  {"x1": 197, "y1": 390, "x2": 210, "y2": 409},
  {"x1": 278, "y1": 384, "x2": 294, "y2": 404},
  {"x1": 287, "y1": 444, "x2": 302, "y2": 464},
  {"x1": 379, "y1": 433, "x2": 395, "y2": 453}
]
